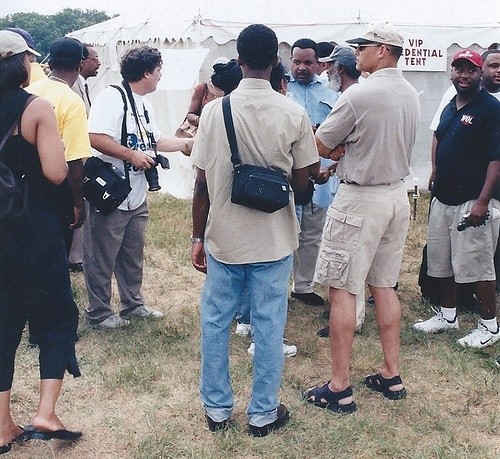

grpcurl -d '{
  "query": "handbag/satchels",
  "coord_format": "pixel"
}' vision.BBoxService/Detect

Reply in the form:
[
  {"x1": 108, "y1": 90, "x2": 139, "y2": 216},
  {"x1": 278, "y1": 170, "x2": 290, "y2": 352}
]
[
  {"x1": 174, "y1": 118, "x2": 198, "y2": 156},
  {"x1": 231, "y1": 164, "x2": 290, "y2": 213},
  {"x1": 82, "y1": 156, "x2": 133, "y2": 215}
]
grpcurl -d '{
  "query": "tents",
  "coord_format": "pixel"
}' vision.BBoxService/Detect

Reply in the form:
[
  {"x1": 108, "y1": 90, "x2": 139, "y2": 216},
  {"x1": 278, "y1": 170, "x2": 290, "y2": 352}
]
[{"x1": 65, "y1": 1, "x2": 500, "y2": 202}]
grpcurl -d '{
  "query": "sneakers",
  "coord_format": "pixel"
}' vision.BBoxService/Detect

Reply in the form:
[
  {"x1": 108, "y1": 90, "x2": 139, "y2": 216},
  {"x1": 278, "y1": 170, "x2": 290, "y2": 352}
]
[
  {"x1": 413, "y1": 304, "x2": 459, "y2": 333},
  {"x1": 235, "y1": 321, "x2": 298, "y2": 359},
  {"x1": 87, "y1": 304, "x2": 165, "y2": 330},
  {"x1": 456, "y1": 322, "x2": 500, "y2": 349}
]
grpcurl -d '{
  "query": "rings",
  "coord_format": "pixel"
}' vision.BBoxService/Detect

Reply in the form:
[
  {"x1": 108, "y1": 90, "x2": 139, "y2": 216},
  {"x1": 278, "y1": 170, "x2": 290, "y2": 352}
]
[{"x1": 80, "y1": 221, "x2": 83, "y2": 223}]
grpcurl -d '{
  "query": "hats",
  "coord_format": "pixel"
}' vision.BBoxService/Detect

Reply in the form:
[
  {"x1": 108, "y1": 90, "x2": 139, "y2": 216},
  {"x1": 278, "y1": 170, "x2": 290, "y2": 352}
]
[
  {"x1": 50, "y1": 37, "x2": 85, "y2": 63},
  {"x1": 317, "y1": 44, "x2": 357, "y2": 67},
  {"x1": 212, "y1": 56, "x2": 231, "y2": 72},
  {"x1": 345, "y1": 21, "x2": 404, "y2": 49},
  {"x1": 6, "y1": 27, "x2": 41, "y2": 53},
  {"x1": 450, "y1": 50, "x2": 483, "y2": 70},
  {"x1": 0, "y1": 29, "x2": 41, "y2": 60}
]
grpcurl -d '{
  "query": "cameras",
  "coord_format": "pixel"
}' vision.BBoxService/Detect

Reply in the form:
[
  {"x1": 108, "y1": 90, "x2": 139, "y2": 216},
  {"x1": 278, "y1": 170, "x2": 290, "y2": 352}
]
[{"x1": 144, "y1": 154, "x2": 169, "y2": 192}]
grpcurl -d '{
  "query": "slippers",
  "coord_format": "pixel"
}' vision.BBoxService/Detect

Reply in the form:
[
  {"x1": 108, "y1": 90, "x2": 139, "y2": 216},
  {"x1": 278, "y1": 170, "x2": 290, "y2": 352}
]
[
  {"x1": 0, "y1": 425, "x2": 34, "y2": 454},
  {"x1": 29, "y1": 428, "x2": 82, "y2": 442}
]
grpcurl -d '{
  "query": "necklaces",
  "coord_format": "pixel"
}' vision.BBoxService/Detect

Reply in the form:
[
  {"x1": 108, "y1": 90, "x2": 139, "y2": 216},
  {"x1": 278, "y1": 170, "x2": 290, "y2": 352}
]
[{"x1": 50, "y1": 76, "x2": 67, "y2": 84}]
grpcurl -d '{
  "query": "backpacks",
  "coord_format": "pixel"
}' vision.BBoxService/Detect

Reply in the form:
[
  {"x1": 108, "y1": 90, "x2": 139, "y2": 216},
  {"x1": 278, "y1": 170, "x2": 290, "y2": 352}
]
[{"x1": 0, "y1": 94, "x2": 37, "y2": 231}]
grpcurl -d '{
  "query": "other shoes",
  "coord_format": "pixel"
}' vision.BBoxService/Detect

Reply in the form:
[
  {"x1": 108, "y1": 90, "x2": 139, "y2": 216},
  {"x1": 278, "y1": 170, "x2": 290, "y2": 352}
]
[
  {"x1": 28, "y1": 333, "x2": 80, "y2": 347},
  {"x1": 466, "y1": 294, "x2": 481, "y2": 312},
  {"x1": 290, "y1": 291, "x2": 326, "y2": 306},
  {"x1": 317, "y1": 309, "x2": 331, "y2": 336},
  {"x1": 206, "y1": 402, "x2": 290, "y2": 437},
  {"x1": 368, "y1": 281, "x2": 398, "y2": 304},
  {"x1": 69, "y1": 262, "x2": 84, "y2": 272}
]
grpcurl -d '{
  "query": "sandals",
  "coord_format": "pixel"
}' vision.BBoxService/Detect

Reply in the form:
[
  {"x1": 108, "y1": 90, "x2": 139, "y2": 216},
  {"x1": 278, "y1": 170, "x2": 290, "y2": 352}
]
[
  {"x1": 302, "y1": 378, "x2": 357, "y2": 415},
  {"x1": 364, "y1": 370, "x2": 407, "y2": 400}
]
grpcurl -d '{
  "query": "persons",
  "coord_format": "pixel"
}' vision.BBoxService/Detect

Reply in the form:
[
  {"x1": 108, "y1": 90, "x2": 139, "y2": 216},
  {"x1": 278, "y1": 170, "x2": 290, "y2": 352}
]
[
  {"x1": 0, "y1": 28, "x2": 83, "y2": 453},
  {"x1": 22, "y1": 37, "x2": 92, "y2": 350},
  {"x1": 186, "y1": 39, "x2": 364, "y2": 359},
  {"x1": 412, "y1": 48, "x2": 500, "y2": 352},
  {"x1": 189, "y1": 24, "x2": 318, "y2": 436},
  {"x1": 303, "y1": 22, "x2": 419, "y2": 414},
  {"x1": 69, "y1": 44, "x2": 101, "y2": 271},
  {"x1": 5, "y1": 27, "x2": 48, "y2": 85},
  {"x1": 83, "y1": 47, "x2": 195, "y2": 329}
]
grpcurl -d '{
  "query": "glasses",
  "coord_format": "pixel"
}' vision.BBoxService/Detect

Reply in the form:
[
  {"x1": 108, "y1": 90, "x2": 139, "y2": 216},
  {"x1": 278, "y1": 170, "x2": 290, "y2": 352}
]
[
  {"x1": 282, "y1": 75, "x2": 291, "y2": 84},
  {"x1": 357, "y1": 43, "x2": 390, "y2": 55},
  {"x1": 83, "y1": 56, "x2": 99, "y2": 64}
]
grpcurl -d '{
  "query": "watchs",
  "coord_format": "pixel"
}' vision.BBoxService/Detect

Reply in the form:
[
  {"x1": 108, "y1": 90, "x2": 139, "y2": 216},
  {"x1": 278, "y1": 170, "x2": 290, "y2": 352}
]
[
  {"x1": 190, "y1": 235, "x2": 203, "y2": 243},
  {"x1": 328, "y1": 167, "x2": 334, "y2": 176}
]
